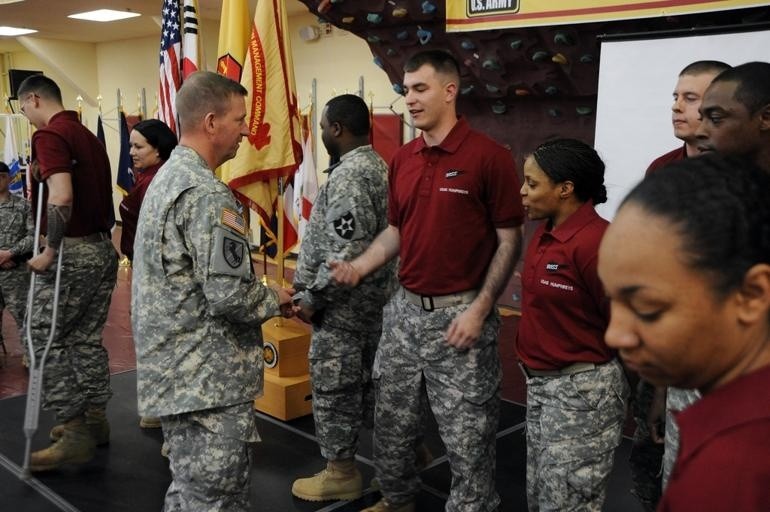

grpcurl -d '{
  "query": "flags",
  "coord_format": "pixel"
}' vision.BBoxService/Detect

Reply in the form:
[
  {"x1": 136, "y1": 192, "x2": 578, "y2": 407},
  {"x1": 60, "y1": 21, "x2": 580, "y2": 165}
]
[
  {"x1": 21, "y1": 124, "x2": 33, "y2": 202},
  {"x1": 156, "y1": 1, "x2": 321, "y2": 264},
  {"x1": 79, "y1": 109, "x2": 144, "y2": 201},
  {"x1": 2, "y1": 119, "x2": 24, "y2": 198}
]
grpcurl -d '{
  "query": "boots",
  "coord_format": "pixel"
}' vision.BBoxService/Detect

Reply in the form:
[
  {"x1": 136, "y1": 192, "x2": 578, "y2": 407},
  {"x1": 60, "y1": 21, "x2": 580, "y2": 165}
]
[
  {"x1": 28, "y1": 421, "x2": 92, "y2": 472},
  {"x1": 52, "y1": 420, "x2": 113, "y2": 448},
  {"x1": 291, "y1": 455, "x2": 363, "y2": 502},
  {"x1": 361, "y1": 494, "x2": 419, "y2": 512}
]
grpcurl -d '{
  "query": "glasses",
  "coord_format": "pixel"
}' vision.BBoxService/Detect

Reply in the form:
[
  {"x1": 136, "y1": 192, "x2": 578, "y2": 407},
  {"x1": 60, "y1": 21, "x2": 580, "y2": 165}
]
[{"x1": 17, "y1": 90, "x2": 40, "y2": 115}]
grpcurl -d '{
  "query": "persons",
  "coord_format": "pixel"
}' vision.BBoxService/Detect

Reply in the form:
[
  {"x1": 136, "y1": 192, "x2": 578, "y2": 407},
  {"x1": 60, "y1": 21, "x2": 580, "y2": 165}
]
[
  {"x1": 514, "y1": 136, "x2": 629, "y2": 512},
  {"x1": 1, "y1": 161, "x2": 38, "y2": 373},
  {"x1": 646, "y1": 61, "x2": 770, "y2": 501},
  {"x1": 631, "y1": 61, "x2": 733, "y2": 512},
  {"x1": 288, "y1": 94, "x2": 391, "y2": 504},
  {"x1": 118, "y1": 119, "x2": 178, "y2": 430},
  {"x1": 18, "y1": 76, "x2": 121, "y2": 471},
  {"x1": 597, "y1": 149, "x2": 770, "y2": 512},
  {"x1": 327, "y1": 47, "x2": 524, "y2": 512},
  {"x1": 126, "y1": 69, "x2": 300, "y2": 512}
]
